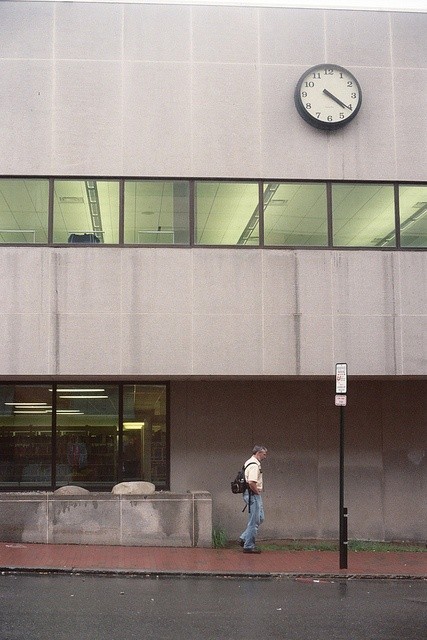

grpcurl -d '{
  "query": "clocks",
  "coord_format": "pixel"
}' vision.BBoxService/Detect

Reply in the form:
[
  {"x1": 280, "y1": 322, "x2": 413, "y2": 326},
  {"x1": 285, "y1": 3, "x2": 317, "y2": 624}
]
[{"x1": 293, "y1": 62, "x2": 362, "y2": 131}]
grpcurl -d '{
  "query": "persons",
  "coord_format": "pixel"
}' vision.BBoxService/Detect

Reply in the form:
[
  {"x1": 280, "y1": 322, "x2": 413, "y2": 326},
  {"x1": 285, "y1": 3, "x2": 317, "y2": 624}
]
[{"x1": 239, "y1": 444, "x2": 267, "y2": 553}]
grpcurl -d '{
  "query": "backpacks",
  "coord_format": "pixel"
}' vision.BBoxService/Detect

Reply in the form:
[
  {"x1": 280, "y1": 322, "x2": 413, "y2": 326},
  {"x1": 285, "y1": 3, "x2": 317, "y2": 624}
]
[{"x1": 231, "y1": 462, "x2": 257, "y2": 513}]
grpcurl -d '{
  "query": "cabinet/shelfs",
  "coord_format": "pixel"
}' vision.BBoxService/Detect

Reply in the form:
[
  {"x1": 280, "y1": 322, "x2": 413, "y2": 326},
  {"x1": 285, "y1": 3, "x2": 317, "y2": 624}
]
[
  {"x1": 142, "y1": 414, "x2": 168, "y2": 486},
  {"x1": 0, "y1": 425, "x2": 117, "y2": 489}
]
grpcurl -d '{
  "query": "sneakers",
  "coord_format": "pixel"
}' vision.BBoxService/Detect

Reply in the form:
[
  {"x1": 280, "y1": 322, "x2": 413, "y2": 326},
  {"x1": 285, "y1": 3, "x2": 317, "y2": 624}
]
[{"x1": 243, "y1": 546, "x2": 261, "y2": 554}]
